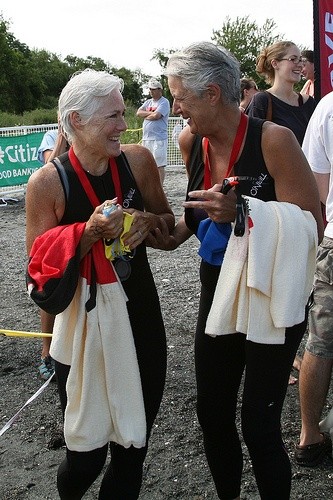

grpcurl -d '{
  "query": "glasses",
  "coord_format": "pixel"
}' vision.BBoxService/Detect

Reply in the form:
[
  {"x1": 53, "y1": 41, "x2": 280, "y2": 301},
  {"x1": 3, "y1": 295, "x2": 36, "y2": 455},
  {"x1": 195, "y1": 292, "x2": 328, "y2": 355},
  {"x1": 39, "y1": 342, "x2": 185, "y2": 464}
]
[
  {"x1": 220, "y1": 177, "x2": 239, "y2": 194},
  {"x1": 102, "y1": 203, "x2": 117, "y2": 216},
  {"x1": 250, "y1": 85, "x2": 259, "y2": 91},
  {"x1": 275, "y1": 56, "x2": 303, "y2": 64}
]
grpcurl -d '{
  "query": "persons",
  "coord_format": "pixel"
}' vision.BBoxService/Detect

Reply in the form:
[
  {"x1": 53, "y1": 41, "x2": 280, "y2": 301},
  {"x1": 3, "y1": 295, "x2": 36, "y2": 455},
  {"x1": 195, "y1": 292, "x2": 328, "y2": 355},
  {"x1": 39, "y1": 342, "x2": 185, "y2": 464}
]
[
  {"x1": 145, "y1": 41, "x2": 324, "y2": 500},
  {"x1": 237, "y1": 77, "x2": 260, "y2": 113},
  {"x1": 25, "y1": 70, "x2": 175, "y2": 500},
  {"x1": 37, "y1": 128, "x2": 67, "y2": 381},
  {"x1": 244, "y1": 41, "x2": 316, "y2": 385},
  {"x1": 294, "y1": 91, "x2": 333, "y2": 466},
  {"x1": 299, "y1": 48, "x2": 315, "y2": 100},
  {"x1": 136, "y1": 80, "x2": 170, "y2": 187}
]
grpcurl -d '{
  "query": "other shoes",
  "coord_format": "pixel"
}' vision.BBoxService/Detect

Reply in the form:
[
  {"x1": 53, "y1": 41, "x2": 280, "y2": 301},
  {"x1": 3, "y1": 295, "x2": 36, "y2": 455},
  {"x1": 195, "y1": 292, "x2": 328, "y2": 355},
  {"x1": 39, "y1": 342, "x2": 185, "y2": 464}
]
[{"x1": 295, "y1": 432, "x2": 333, "y2": 466}]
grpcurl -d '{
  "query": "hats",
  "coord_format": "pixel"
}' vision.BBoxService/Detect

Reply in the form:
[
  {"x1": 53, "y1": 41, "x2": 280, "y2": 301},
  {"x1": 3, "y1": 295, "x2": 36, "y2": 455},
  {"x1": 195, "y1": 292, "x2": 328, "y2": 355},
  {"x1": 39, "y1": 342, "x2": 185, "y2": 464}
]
[{"x1": 145, "y1": 81, "x2": 164, "y2": 90}]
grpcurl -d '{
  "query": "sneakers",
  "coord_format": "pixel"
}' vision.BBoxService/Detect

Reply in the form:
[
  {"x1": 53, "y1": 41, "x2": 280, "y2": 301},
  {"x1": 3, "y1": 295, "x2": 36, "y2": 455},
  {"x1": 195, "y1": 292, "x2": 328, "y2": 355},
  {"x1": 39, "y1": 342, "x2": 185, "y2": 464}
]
[{"x1": 39, "y1": 356, "x2": 56, "y2": 381}]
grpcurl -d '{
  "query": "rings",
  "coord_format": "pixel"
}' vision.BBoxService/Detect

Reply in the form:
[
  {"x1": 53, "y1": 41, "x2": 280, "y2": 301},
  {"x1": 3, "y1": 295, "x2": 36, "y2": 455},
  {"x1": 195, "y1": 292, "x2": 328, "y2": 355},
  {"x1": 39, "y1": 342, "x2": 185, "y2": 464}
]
[{"x1": 136, "y1": 229, "x2": 143, "y2": 237}]
